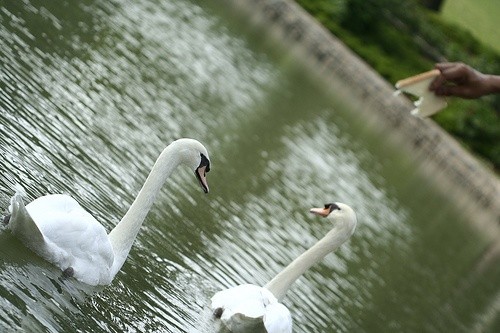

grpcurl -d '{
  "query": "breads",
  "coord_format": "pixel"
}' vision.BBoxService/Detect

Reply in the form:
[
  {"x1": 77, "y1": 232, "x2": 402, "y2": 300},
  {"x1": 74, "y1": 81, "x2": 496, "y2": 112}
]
[{"x1": 395, "y1": 69, "x2": 449, "y2": 119}]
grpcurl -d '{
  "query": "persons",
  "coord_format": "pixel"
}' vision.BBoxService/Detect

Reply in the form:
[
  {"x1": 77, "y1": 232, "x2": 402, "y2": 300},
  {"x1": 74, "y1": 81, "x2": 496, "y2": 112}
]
[{"x1": 429, "y1": 62, "x2": 499, "y2": 99}]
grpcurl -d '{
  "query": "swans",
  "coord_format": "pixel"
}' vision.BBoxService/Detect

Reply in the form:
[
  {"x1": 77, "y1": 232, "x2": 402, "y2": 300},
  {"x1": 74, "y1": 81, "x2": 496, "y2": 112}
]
[
  {"x1": 3, "y1": 137, "x2": 212, "y2": 287},
  {"x1": 210, "y1": 202, "x2": 357, "y2": 333}
]
